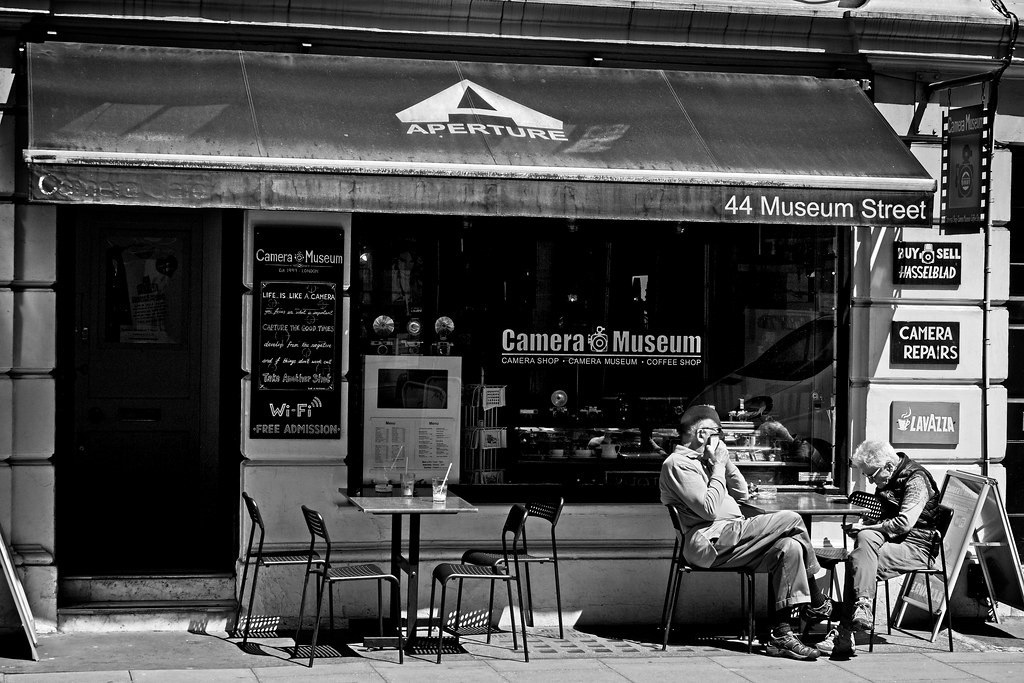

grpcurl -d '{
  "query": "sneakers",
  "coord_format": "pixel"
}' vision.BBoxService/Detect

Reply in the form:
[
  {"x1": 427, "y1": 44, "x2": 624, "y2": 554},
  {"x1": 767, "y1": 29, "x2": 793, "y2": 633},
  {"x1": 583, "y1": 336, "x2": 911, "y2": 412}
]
[
  {"x1": 852, "y1": 600, "x2": 873, "y2": 629},
  {"x1": 815, "y1": 627, "x2": 856, "y2": 654},
  {"x1": 800, "y1": 595, "x2": 845, "y2": 623},
  {"x1": 767, "y1": 628, "x2": 820, "y2": 659}
]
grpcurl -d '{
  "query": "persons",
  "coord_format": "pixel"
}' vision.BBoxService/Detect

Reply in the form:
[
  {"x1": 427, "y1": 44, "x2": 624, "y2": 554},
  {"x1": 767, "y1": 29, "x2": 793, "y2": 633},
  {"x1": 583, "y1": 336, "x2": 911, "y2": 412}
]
[
  {"x1": 754, "y1": 419, "x2": 826, "y2": 488},
  {"x1": 813, "y1": 437, "x2": 940, "y2": 658},
  {"x1": 658, "y1": 405, "x2": 843, "y2": 661}
]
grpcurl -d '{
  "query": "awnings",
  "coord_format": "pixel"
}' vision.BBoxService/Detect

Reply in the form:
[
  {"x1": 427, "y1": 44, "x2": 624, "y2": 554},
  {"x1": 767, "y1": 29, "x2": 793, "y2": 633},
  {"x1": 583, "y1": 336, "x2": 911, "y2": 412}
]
[{"x1": 18, "y1": 39, "x2": 938, "y2": 230}]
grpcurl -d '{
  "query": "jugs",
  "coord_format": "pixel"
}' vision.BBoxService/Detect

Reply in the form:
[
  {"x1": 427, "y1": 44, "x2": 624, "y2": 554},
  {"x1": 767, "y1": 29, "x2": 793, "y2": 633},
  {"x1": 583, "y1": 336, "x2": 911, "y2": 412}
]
[{"x1": 601, "y1": 444, "x2": 621, "y2": 458}]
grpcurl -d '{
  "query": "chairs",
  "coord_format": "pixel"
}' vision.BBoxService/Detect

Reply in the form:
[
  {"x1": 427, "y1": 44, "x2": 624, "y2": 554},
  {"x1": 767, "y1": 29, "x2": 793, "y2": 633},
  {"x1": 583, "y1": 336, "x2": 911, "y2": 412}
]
[
  {"x1": 232, "y1": 491, "x2": 565, "y2": 668},
  {"x1": 659, "y1": 502, "x2": 954, "y2": 654}
]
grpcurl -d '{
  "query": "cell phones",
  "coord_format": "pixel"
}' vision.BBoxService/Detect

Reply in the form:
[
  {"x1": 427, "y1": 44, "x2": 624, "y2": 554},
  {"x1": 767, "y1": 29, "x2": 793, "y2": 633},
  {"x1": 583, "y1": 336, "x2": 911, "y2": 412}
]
[{"x1": 708, "y1": 436, "x2": 720, "y2": 465}]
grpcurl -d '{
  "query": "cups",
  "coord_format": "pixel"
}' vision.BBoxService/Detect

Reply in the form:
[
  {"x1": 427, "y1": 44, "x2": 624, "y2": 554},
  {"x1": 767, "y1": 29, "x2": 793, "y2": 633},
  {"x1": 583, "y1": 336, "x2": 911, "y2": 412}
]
[
  {"x1": 550, "y1": 449, "x2": 563, "y2": 456},
  {"x1": 432, "y1": 477, "x2": 448, "y2": 502},
  {"x1": 400, "y1": 473, "x2": 415, "y2": 496},
  {"x1": 375, "y1": 469, "x2": 390, "y2": 489}
]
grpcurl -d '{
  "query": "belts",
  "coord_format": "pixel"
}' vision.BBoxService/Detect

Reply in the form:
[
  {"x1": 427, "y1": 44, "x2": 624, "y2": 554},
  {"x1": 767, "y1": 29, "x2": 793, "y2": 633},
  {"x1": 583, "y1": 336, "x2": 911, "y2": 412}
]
[{"x1": 688, "y1": 519, "x2": 714, "y2": 537}]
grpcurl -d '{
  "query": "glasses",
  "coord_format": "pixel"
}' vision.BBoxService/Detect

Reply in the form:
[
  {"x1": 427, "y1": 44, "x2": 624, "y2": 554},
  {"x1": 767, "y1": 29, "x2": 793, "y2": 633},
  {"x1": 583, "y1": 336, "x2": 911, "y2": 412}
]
[
  {"x1": 696, "y1": 424, "x2": 722, "y2": 435},
  {"x1": 861, "y1": 466, "x2": 882, "y2": 480}
]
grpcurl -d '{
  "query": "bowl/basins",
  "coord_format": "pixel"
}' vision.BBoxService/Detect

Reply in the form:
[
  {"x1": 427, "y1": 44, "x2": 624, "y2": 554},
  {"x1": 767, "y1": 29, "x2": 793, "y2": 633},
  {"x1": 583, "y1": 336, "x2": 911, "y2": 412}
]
[{"x1": 576, "y1": 448, "x2": 588, "y2": 456}]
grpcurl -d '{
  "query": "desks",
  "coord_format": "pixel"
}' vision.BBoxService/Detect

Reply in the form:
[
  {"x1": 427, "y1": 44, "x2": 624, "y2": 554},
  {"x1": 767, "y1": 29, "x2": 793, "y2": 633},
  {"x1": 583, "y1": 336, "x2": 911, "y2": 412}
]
[
  {"x1": 739, "y1": 492, "x2": 872, "y2": 652},
  {"x1": 338, "y1": 487, "x2": 478, "y2": 655}
]
